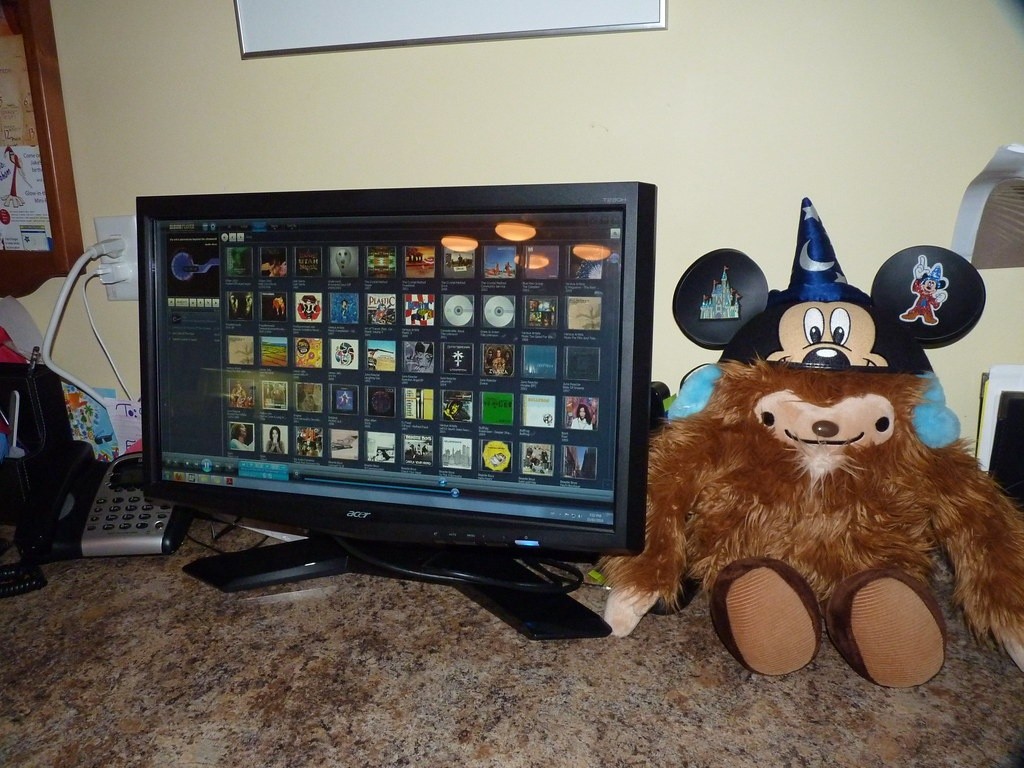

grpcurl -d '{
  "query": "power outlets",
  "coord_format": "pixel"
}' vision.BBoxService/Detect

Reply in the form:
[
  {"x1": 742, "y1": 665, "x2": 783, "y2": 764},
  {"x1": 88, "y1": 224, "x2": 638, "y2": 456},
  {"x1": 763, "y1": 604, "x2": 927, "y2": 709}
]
[{"x1": 93, "y1": 215, "x2": 140, "y2": 302}]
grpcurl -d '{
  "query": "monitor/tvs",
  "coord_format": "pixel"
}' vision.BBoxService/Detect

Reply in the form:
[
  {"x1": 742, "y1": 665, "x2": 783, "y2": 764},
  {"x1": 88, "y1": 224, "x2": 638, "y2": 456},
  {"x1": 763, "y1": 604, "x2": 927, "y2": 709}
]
[{"x1": 134, "y1": 182, "x2": 656, "y2": 639}]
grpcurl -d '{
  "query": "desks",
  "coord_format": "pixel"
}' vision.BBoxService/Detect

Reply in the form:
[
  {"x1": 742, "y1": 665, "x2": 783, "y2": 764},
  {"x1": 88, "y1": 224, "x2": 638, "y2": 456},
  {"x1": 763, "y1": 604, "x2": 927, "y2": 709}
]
[{"x1": 0, "y1": 516, "x2": 1024, "y2": 767}]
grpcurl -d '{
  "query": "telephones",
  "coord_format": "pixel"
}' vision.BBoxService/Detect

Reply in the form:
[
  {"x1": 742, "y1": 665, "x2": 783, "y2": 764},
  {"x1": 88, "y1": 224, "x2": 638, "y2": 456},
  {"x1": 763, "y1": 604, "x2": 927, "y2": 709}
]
[{"x1": 15, "y1": 441, "x2": 190, "y2": 557}]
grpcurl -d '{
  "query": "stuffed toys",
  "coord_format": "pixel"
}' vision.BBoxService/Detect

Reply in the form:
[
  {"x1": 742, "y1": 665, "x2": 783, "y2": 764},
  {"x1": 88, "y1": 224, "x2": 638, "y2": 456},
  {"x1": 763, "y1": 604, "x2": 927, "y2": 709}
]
[{"x1": 596, "y1": 198, "x2": 1024, "y2": 685}]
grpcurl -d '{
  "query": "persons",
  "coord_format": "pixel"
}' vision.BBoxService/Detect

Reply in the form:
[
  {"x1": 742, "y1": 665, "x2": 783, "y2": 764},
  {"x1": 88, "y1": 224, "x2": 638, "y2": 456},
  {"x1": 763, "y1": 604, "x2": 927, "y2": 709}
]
[
  {"x1": 229, "y1": 423, "x2": 323, "y2": 460},
  {"x1": 226, "y1": 247, "x2": 602, "y2": 379},
  {"x1": 330, "y1": 382, "x2": 601, "y2": 484}
]
